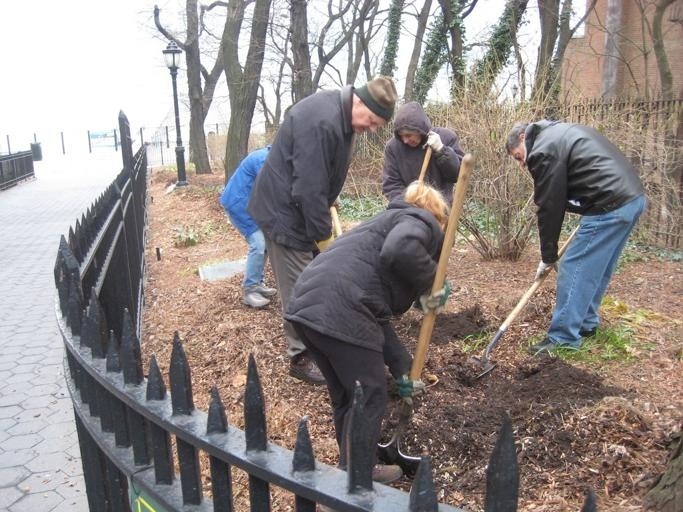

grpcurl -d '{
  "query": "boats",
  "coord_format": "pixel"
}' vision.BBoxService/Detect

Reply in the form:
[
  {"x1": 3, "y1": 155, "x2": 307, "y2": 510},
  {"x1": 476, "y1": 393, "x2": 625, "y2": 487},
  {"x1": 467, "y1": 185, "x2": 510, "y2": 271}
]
[{"x1": 90, "y1": 131, "x2": 137, "y2": 147}]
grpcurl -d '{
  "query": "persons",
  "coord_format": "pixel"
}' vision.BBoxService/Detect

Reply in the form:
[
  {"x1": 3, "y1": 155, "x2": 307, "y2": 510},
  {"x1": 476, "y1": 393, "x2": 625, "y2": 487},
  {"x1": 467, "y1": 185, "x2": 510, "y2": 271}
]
[
  {"x1": 217, "y1": 144, "x2": 277, "y2": 309},
  {"x1": 244, "y1": 74, "x2": 398, "y2": 387},
  {"x1": 378, "y1": 99, "x2": 466, "y2": 319},
  {"x1": 501, "y1": 116, "x2": 645, "y2": 355},
  {"x1": 282, "y1": 175, "x2": 454, "y2": 488}
]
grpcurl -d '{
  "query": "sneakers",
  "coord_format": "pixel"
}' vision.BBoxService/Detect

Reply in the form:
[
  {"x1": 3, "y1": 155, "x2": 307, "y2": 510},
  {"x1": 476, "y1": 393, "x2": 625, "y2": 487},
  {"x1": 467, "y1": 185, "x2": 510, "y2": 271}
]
[
  {"x1": 289, "y1": 354, "x2": 326, "y2": 384},
  {"x1": 240, "y1": 282, "x2": 277, "y2": 308},
  {"x1": 528, "y1": 324, "x2": 597, "y2": 356},
  {"x1": 338, "y1": 463, "x2": 403, "y2": 484}
]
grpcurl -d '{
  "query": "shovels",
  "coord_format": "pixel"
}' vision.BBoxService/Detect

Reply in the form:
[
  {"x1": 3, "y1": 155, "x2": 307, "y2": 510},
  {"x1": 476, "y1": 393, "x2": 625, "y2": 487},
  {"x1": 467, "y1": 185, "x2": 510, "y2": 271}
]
[
  {"x1": 460, "y1": 223, "x2": 583, "y2": 383},
  {"x1": 376, "y1": 152, "x2": 476, "y2": 483}
]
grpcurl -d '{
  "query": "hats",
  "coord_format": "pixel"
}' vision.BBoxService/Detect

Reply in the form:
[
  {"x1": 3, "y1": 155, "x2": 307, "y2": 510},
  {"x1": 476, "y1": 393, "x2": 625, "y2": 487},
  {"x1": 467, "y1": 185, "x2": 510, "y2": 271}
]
[{"x1": 353, "y1": 73, "x2": 398, "y2": 123}]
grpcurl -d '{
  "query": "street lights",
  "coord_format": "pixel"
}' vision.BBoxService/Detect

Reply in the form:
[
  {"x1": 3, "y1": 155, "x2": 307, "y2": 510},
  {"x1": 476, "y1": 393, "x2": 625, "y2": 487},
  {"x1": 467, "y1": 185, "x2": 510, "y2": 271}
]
[{"x1": 163, "y1": 39, "x2": 191, "y2": 187}]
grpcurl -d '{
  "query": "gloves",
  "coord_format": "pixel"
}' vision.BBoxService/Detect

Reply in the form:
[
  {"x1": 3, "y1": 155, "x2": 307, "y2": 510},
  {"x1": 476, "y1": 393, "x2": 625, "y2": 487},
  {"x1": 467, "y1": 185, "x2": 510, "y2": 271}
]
[
  {"x1": 533, "y1": 259, "x2": 559, "y2": 281},
  {"x1": 419, "y1": 278, "x2": 451, "y2": 315},
  {"x1": 393, "y1": 373, "x2": 426, "y2": 406},
  {"x1": 425, "y1": 130, "x2": 444, "y2": 153}
]
